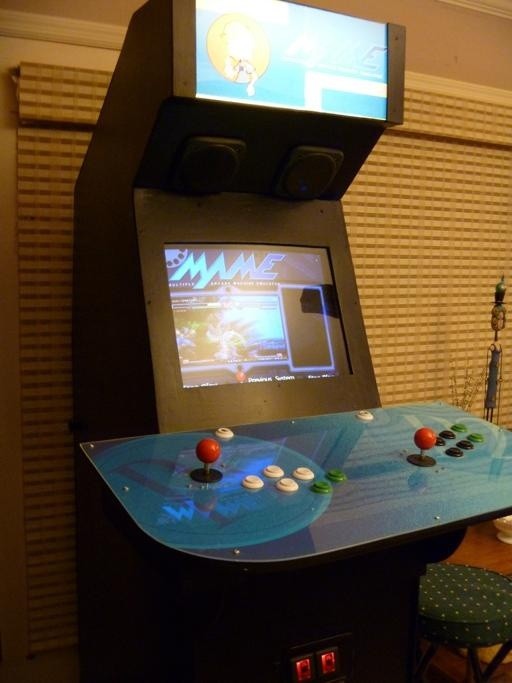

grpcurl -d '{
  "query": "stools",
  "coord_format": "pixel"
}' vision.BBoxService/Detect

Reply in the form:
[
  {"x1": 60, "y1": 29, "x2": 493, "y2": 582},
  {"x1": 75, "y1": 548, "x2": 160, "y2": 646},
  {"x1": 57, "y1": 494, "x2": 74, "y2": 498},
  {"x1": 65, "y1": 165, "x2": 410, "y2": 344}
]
[{"x1": 409, "y1": 561, "x2": 511, "y2": 680}]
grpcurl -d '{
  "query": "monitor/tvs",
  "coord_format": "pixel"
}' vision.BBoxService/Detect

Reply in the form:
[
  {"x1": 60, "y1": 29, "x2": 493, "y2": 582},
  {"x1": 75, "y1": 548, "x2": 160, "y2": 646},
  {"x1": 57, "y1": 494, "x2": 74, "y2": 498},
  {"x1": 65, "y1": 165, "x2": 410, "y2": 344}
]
[{"x1": 163, "y1": 242, "x2": 352, "y2": 389}]
